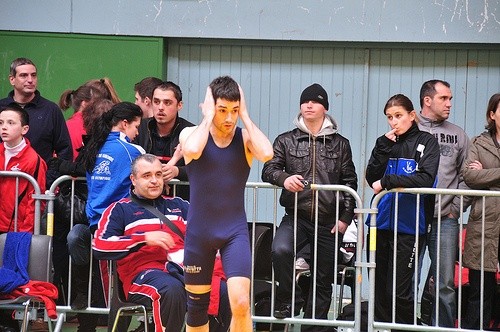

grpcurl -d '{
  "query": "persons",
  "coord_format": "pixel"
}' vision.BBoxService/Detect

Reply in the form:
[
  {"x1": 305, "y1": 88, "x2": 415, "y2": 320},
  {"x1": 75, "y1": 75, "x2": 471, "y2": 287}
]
[
  {"x1": 61, "y1": 84, "x2": 102, "y2": 163},
  {"x1": 46, "y1": 99, "x2": 115, "y2": 331},
  {"x1": 0, "y1": 103, "x2": 47, "y2": 332},
  {"x1": 134, "y1": 77, "x2": 165, "y2": 118},
  {"x1": 178, "y1": 75, "x2": 274, "y2": 332},
  {"x1": 79, "y1": 102, "x2": 182, "y2": 332},
  {"x1": 132, "y1": 81, "x2": 197, "y2": 203},
  {"x1": 0, "y1": 57, "x2": 73, "y2": 318},
  {"x1": 413, "y1": 79, "x2": 480, "y2": 332},
  {"x1": 261, "y1": 82, "x2": 358, "y2": 332},
  {"x1": 86, "y1": 79, "x2": 122, "y2": 106},
  {"x1": 366, "y1": 94, "x2": 440, "y2": 331},
  {"x1": 92, "y1": 154, "x2": 231, "y2": 332},
  {"x1": 464, "y1": 93, "x2": 500, "y2": 332}
]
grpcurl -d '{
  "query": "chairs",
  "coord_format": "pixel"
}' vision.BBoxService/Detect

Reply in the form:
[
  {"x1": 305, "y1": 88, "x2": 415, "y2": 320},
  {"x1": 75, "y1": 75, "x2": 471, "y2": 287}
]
[
  {"x1": 250, "y1": 220, "x2": 364, "y2": 328},
  {"x1": 107, "y1": 258, "x2": 156, "y2": 332},
  {"x1": 0, "y1": 234, "x2": 52, "y2": 332}
]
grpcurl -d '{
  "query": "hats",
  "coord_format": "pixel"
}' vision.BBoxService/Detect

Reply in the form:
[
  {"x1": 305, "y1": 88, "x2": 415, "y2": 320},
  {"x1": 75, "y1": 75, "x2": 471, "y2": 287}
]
[{"x1": 299, "y1": 83, "x2": 329, "y2": 110}]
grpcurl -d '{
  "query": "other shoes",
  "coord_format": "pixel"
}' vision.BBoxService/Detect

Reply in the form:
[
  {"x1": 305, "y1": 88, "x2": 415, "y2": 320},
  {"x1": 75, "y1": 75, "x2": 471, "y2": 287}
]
[
  {"x1": 71, "y1": 283, "x2": 96, "y2": 310},
  {"x1": 274, "y1": 303, "x2": 300, "y2": 318}
]
